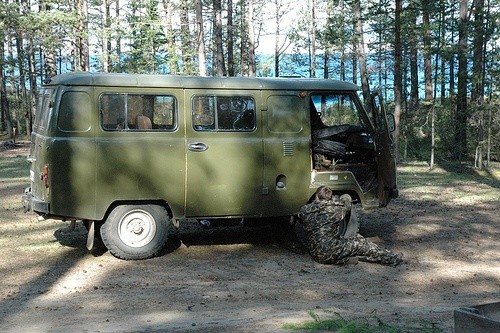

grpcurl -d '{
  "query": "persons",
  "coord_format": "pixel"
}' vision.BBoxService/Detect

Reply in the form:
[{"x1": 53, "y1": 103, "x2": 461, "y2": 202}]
[{"x1": 294, "y1": 185, "x2": 404, "y2": 269}]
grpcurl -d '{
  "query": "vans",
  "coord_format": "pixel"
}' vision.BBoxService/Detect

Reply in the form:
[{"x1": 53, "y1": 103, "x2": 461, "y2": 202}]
[{"x1": 22, "y1": 71, "x2": 398, "y2": 260}]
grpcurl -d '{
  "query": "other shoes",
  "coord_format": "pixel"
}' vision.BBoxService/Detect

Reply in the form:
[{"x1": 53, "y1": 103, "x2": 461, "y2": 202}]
[{"x1": 391, "y1": 253, "x2": 404, "y2": 265}]
